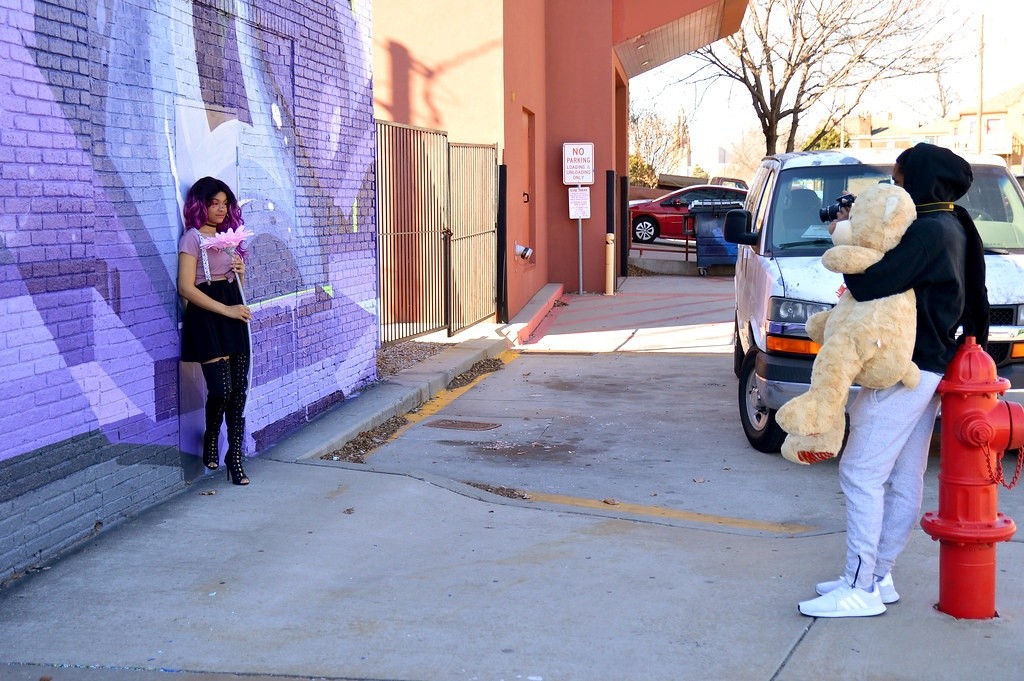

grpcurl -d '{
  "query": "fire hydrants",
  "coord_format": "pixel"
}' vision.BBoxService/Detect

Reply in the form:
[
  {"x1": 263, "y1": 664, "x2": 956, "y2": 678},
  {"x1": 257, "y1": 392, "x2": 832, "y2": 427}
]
[{"x1": 920, "y1": 333, "x2": 1023, "y2": 619}]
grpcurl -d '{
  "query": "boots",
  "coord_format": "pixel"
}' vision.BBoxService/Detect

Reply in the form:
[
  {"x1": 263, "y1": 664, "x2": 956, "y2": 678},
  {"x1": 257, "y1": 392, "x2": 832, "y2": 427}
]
[
  {"x1": 200, "y1": 357, "x2": 233, "y2": 470},
  {"x1": 224, "y1": 356, "x2": 251, "y2": 485}
]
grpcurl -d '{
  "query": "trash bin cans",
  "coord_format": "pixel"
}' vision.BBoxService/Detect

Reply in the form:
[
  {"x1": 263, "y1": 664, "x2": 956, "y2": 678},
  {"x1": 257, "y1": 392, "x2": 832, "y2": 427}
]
[{"x1": 687, "y1": 199, "x2": 745, "y2": 277}]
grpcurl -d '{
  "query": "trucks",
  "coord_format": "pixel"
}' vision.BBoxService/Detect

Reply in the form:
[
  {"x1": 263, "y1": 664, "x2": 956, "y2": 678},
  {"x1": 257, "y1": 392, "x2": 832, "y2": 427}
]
[{"x1": 630, "y1": 177, "x2": 750, "y2": 201}]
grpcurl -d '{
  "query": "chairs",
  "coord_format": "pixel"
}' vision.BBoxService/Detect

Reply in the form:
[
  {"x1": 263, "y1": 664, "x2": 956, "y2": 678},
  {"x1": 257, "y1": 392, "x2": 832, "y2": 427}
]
[{"x1": 783, "y1": 189, "x2": 821, "y2": 229}]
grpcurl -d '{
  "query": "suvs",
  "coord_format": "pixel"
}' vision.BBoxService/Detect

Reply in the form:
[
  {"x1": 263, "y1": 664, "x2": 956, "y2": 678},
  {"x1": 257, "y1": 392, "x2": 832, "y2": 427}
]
[{"x1": 722, "y1": 146, "x2": 1023, "y2": 454}]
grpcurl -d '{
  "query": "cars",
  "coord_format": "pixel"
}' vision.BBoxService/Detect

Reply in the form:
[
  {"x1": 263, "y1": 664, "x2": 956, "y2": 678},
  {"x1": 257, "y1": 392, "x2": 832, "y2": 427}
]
[{"x1": 630, "y1": 185, "x2": 748, "y2": 244}]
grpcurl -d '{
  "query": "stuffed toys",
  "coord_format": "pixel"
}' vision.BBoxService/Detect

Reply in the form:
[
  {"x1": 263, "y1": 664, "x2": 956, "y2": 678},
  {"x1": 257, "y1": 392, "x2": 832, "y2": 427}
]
[{"x1": 775, "y1": 183, "x2": 921, "y2": 465}]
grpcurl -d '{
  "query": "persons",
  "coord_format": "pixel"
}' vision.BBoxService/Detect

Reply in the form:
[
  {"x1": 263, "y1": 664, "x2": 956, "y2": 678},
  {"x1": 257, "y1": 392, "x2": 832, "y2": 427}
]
[
  {"x1": 178, "y1": 176, "x2": 252, "y2": 485},
  {"x1": 798, "y1": 142, "x2": 966, "y2": 617}
]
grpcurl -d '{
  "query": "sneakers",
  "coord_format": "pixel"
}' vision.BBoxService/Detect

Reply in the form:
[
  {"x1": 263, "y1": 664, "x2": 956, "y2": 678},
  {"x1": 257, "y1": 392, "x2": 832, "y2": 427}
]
[{"x1": 797, "y1": 568, "x2": 900, "y2": 618}]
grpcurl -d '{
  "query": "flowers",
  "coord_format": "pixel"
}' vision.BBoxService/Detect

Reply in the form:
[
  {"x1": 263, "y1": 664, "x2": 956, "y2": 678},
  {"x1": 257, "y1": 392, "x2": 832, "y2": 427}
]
[{"x1": 199, "y1": 226, "x2": 253, "y2": 418}]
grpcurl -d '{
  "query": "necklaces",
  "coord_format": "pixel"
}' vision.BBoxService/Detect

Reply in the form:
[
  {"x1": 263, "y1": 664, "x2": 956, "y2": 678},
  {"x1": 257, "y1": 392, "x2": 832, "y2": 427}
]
[{"x1": 205, "y1": 223, "x2": 217, "y2": 227}]
